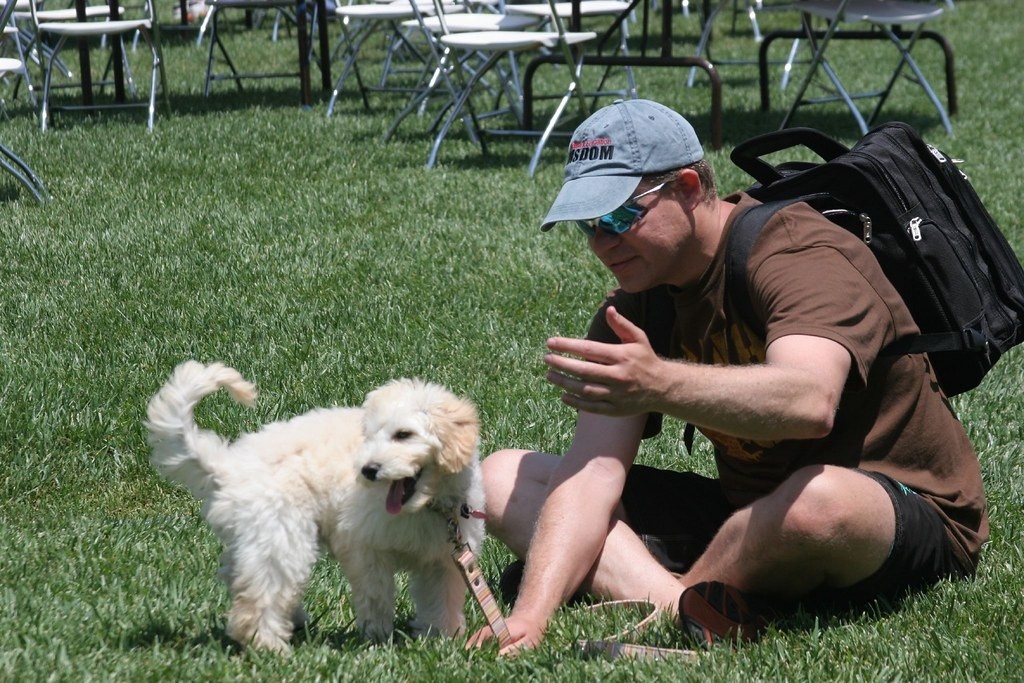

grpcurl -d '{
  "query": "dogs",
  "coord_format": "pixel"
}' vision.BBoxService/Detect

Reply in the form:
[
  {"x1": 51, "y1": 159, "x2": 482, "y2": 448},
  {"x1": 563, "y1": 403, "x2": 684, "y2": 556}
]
[{"x1": 143, "y1": 358, "x2": 486, "y2": 660}]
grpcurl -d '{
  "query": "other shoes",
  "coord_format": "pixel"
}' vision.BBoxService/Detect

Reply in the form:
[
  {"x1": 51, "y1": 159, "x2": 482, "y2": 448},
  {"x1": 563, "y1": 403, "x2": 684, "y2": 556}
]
[
  {"x1": 680, "y1": 580, "x2": 789, "y2": 652},
  {"x1": 500, "y1": 558, "x2": 597, "y2": 611}
]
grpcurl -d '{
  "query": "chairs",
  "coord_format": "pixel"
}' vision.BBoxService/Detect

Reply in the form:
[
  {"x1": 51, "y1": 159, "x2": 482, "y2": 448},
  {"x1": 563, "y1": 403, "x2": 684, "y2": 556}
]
[{"x1": 0, "y1": 0, "x2": 959, "y2": 205}]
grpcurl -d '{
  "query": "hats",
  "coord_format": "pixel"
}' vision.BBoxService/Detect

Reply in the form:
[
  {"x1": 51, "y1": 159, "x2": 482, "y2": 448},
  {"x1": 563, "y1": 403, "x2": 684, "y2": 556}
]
[{"x1": 540, "y1": 99, "x2": 705, "y2": 232}]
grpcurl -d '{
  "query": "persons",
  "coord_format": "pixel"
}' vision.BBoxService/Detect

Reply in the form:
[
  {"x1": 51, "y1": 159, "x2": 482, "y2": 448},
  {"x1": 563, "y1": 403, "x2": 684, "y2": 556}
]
[{"x1": 465, "y1": 98, "x2": 988, "y2": 655}]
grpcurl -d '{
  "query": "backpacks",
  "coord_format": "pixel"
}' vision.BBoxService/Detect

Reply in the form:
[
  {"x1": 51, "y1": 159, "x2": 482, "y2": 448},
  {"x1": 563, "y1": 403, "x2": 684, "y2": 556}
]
[{"x1": 652, "y1": 120, "x2": 1024, "y2": 397}]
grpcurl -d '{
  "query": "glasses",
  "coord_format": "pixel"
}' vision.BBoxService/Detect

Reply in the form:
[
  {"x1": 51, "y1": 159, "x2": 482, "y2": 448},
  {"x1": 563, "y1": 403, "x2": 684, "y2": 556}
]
[{"x1": 574, "y1": 174, "x2": 681, "y2": 237}]
